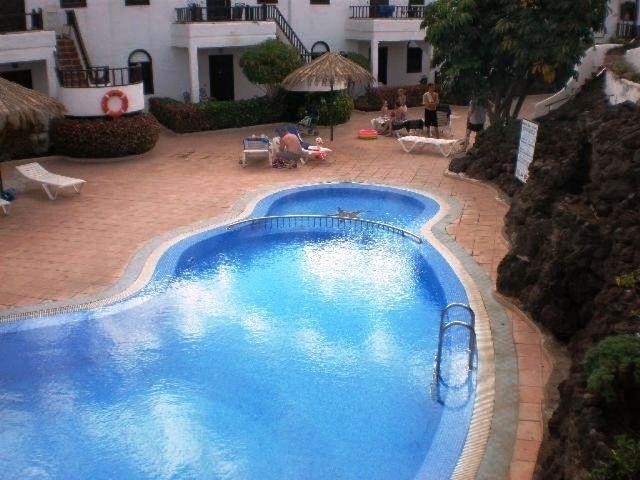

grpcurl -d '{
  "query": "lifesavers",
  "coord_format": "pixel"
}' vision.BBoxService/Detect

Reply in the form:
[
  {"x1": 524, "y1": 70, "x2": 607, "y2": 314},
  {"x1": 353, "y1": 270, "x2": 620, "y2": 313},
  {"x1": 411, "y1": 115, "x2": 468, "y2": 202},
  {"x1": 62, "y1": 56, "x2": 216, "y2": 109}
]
[
  {"x1": 359, "y1": 128, "x2": 378, "y2": 140},
  {"x1": 101, "y1": 90, "x2": 128, "y2": 119}
]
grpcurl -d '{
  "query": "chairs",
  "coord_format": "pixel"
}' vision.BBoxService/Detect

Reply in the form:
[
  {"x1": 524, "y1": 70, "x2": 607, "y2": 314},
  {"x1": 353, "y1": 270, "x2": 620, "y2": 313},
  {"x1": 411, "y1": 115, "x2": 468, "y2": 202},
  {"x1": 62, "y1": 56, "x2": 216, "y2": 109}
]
[
  {"x1": 398, "y1": 135, "x2": 470, "y2": 156},
  {"x1": 0, "y1": 197, "x2": 10, "y2": 216},
  {"x1": 15, "y1": 161, "x2": 87, "y2": 201},
  {"x1": 241, "y1": 136, "x2": 274, "y2": 167},
  {"x1": 435, "y1": 103, "x2": 458, "y2": 136},
  {"x1": 369, "y1": 116, "x2": 427, "y2": 136},
  {"x1": 272, "y1": 124, "x2": 333, "y2": 164},
  {"x1": 88, "y1": 64, "x2": 113, "y2": 88}
]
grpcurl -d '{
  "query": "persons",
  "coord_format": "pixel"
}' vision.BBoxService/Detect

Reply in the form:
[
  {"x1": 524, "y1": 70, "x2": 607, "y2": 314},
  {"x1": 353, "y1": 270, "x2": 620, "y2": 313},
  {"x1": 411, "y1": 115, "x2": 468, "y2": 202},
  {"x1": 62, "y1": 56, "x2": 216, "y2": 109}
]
[
  {"x1": 376, "y1": 84, "x2": 409, "y2": 136},
  {"x1": 276, "y1": 123, "x2": 323, "y2": 151},
  {"x1": 422, "y1": 84, "x2": 440, "y2": 139},
  {"x1": 272, "y1": 128, "x2": 302, "y2": 167},
  {"x1": 457, "y1": 86, "x2": 495, "y2": 154}
]
